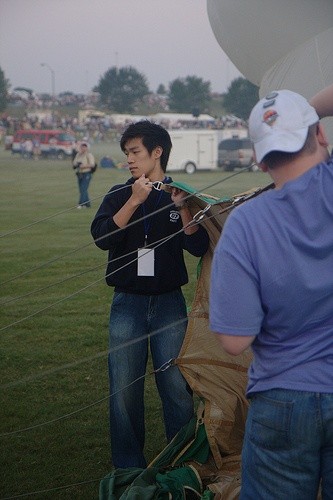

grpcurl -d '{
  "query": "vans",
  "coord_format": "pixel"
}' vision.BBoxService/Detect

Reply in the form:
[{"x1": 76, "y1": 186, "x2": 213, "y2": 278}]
[
  {"x1": 217, "y1": 138, "x2": 257, "y2": 172},
  {"x1": 165, "y1": 130, "x2": 219, "y2": 174},
  {"x1": 12, "y1": 130, "x2": 78, "y2": 161}
]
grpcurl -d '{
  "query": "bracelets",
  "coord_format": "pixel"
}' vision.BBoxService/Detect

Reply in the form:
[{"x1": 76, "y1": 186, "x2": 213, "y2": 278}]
[{"x1": 176, "y1": 204, "x2": 189, "y2": 209}]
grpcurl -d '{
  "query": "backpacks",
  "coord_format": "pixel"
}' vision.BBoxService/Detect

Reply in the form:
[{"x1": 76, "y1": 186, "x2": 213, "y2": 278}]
[{"x1": 86, "y1": 152, "x2": 97, "y2": 173}]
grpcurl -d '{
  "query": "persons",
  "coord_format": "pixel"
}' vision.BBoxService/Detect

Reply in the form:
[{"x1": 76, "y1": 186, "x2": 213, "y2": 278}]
[
  {"x1": 73, "y1": 144, "x2": 98, "y2": 208},
  {"x1": 0, "y1": 92, "x2": 246, "y2": 144},
  {"x1": 22, "y1": 133, "x2": 58, "y2": 160},
  {"x1": 91, "y1": 120, "x2": 211, "y2": 470},
  {"x1": 210, "y1": 83, "x2": 333, "y2": 500}
]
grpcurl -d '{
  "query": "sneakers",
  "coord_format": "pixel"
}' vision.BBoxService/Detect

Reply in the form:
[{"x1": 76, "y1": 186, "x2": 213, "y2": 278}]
[
  {"x1": 77, "y1": 205, "x2": 81, "y2": 209},
  {"x1": 83, "y1": 205, "x2": 89, "y2": 208}
]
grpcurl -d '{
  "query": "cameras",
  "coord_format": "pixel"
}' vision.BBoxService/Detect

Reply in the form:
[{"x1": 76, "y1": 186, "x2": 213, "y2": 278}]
[{"x1": 73, "y1": 162, "x2": 82, "y2": 169}]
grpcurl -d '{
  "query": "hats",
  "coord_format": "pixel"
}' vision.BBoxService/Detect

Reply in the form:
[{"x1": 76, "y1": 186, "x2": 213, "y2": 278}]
[{"x1": 246, "y1": 90, "x2": 320, "y2": 164}]
